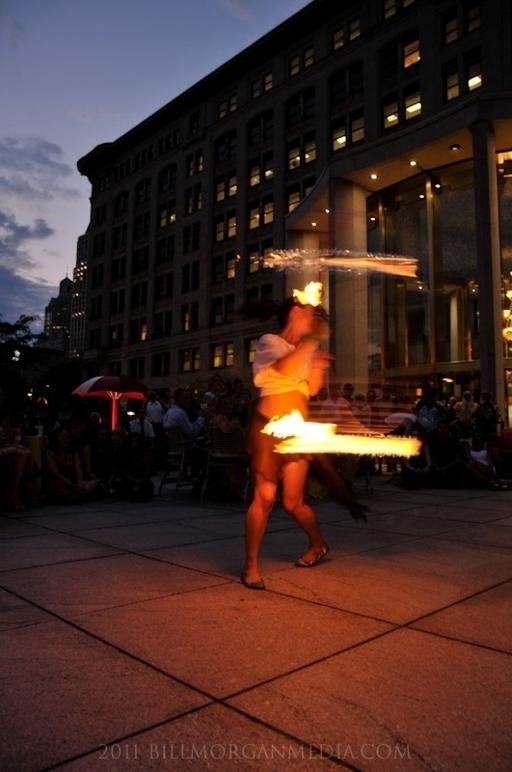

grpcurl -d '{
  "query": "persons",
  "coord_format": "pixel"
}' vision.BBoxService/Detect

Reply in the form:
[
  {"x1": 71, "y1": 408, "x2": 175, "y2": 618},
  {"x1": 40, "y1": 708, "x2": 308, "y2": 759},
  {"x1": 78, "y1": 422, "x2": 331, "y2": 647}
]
[{"x1": 240, "y1": 302, "x2": 331, "y2": 589}]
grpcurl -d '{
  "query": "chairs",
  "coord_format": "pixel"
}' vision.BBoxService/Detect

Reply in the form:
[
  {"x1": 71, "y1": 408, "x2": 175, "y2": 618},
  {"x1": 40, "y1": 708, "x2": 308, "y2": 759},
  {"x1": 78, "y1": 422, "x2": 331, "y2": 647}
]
[{"x1": 157, "y1": 422, "x2": 249, "y2": 509}]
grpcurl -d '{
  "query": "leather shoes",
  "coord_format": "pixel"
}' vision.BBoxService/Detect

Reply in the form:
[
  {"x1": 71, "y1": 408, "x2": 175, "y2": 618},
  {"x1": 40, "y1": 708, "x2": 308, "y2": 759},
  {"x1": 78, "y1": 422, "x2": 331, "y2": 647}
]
[
  {"x1": 241, "y1": 576, "x2": 265, "y2": 588},
  {"x1": 296, "y1": 543, "x2": 327, "y2": 566}
]
